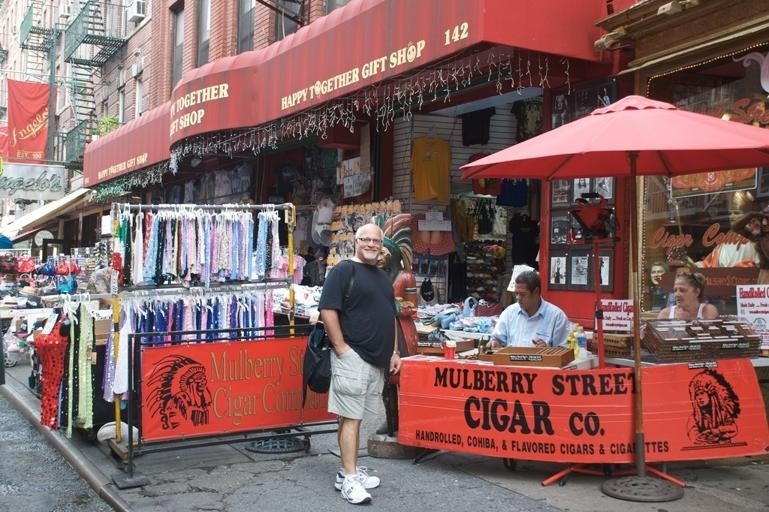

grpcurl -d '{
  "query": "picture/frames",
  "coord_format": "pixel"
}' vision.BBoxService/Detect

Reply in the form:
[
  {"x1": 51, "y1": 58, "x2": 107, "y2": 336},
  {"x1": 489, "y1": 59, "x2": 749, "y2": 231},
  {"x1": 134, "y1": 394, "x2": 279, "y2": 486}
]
[{"x1": 547, "y1": 176, "x2": 617, "y2": 293}]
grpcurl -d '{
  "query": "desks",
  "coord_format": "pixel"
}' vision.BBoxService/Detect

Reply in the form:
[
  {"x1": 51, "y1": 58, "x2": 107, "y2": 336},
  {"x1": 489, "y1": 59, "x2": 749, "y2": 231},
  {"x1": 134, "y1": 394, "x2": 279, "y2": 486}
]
[{"x1": 397, "y1": 352, "x2": 769, "y2": 486}]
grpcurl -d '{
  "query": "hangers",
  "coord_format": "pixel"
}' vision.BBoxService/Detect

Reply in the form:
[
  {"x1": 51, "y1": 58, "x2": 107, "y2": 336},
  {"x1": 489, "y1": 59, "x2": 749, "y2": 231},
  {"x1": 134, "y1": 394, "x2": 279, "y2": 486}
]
[
  {"x1": 131, "y1": 289, "x2": 255, "y2": 320},
  {"x1": 123, "y1": 202, "x2": 279, "y2": 229},
  {"x1": 60, "y1": 292, "x2": 97, "y2": 326}
]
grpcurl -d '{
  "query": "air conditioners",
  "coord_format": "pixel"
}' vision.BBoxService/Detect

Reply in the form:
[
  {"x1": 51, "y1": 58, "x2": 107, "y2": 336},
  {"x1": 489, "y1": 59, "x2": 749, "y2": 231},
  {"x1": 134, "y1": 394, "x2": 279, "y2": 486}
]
[{"x1": 127, "y1": 0, "x2": 146, "y2": 24}]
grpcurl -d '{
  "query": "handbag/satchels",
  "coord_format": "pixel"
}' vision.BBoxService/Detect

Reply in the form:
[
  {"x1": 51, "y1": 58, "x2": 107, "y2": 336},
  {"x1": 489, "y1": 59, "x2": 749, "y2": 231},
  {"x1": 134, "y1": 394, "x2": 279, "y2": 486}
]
[
  {"x1": 302, "y1": 322, "x2": 332, "y2": 409},
  {"x1": 478, "y1": 218, "x2": 507, "y2": 235}
]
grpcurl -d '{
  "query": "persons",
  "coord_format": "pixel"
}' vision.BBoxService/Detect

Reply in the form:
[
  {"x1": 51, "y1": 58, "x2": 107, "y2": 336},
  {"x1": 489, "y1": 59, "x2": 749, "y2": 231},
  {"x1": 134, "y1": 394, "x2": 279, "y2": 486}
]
[
  {"x1": 656, "y1": 271, "x2": 720, "y2": 320},
  {"x1": 486, "y1": 271, "x2": 570, "y2": 349},
  {"x1": 370, "y1": 212, "x2": 419, "y2": 435},
  {"x1": 649, "y1": 260, "x2": 671, "y2": 296},
  {"x1": 680, "y1": 190, "x2": 764, "y2": 269},
  {"x1": 318, "y1": 223, "x2": 401, "y2": 504}
]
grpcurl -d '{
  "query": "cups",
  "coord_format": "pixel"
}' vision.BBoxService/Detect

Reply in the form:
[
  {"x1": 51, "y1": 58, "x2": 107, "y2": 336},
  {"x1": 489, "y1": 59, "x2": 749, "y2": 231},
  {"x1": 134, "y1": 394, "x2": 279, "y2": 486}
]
[{"x1": 440, "y1": 340, "x2": 457, "y2": 360}]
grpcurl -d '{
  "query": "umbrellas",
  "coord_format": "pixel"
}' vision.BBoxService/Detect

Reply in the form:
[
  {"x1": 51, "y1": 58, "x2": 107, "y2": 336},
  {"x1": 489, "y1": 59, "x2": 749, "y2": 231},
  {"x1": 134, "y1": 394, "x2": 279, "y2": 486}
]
[{"x1": 456, "y1": 93, "x2": 769, "y2": 477}]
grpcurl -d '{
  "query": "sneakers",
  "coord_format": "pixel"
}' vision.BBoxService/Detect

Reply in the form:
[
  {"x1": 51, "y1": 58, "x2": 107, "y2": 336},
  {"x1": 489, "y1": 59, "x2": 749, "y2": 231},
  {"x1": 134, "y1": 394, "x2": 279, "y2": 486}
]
[{"x1": 334, "y1": 469, "x2": 380, "y2": 505}]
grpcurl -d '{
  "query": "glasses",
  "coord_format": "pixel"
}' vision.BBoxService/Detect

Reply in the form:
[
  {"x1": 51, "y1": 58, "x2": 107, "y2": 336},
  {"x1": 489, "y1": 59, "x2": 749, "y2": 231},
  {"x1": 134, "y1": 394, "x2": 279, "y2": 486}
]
[{"x1": 357, "y1": 238, "x2": 383, "y2": 245}]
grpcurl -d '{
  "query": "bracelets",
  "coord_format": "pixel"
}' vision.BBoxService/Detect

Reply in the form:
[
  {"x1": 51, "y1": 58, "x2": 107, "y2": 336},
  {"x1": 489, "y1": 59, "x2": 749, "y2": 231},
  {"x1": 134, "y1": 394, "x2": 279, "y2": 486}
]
[{"x1": 392, "y1": 351, "x2": 400, "y2": 355}]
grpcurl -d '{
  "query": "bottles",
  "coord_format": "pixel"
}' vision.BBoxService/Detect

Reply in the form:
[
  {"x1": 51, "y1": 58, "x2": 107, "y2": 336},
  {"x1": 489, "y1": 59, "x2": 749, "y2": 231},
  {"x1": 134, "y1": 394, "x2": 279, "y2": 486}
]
[
  {"x1": 577, "y1": 326, "x2": 588, "y2": 359},
  {"x1": 567, "y1": 330, "x2": 578, "y2": 360}
]
